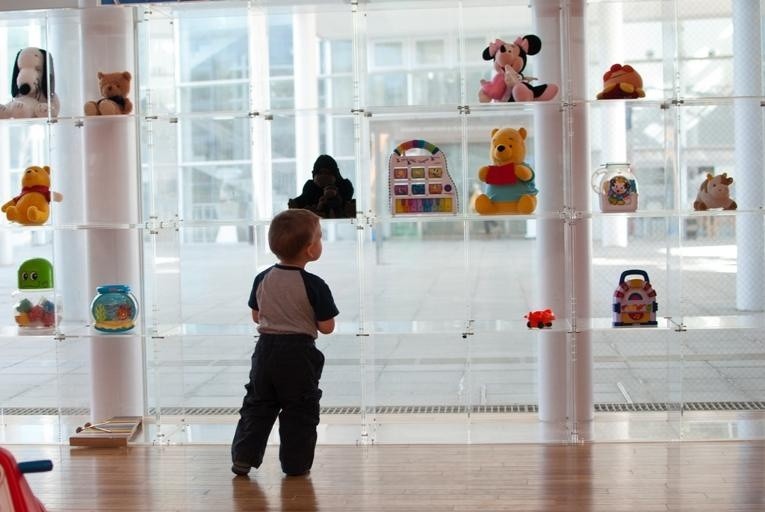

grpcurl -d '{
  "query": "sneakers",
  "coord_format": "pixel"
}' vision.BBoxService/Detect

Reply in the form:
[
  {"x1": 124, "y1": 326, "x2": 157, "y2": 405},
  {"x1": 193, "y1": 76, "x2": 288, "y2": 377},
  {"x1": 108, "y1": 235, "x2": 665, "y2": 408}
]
[{"x1": 232, "y1": 460, "x2": 251, "y2": 475}]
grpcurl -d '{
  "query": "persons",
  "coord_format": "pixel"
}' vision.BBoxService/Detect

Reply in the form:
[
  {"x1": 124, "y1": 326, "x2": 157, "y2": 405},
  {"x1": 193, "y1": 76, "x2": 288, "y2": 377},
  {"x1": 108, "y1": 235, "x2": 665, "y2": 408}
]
[{"x1": 226, "y1": 205, "x2": 341, "y2": 479}]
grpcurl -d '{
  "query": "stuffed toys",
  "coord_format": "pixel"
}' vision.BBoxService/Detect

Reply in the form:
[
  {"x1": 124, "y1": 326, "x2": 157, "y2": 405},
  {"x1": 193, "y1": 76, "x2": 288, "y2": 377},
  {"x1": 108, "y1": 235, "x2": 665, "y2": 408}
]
[
  {"x1": 0, "y1": 164, "x2": 67, "y2": 228},
  {"x1": 595, "y1": 63, "x2": 645, "y2": 100},
  {"x1": 82, "y1": 69, "x2": 134, "y2": 117},
  {"x1": 478, "y1": 33, "x2": 557, "y2": 103},
  {"x1": 473, "y1": 126, "x2": 540, "y2": 217},
  {"x1": 693, "y1": 170, "x2": 738, "y2": 211},
  {"x1": 287, "y1": 154, "x2": 356, "y2": 219},
  {"x1": 0, "y1": 45, "x2": 61, "y2": 118}
]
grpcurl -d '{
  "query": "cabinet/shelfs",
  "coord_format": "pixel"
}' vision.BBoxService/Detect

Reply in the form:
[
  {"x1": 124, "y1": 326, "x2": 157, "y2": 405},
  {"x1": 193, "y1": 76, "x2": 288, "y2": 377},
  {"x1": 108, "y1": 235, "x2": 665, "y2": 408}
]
[{"x1": 1, "y1": 95, "x2": 764, "y2": 337}]
